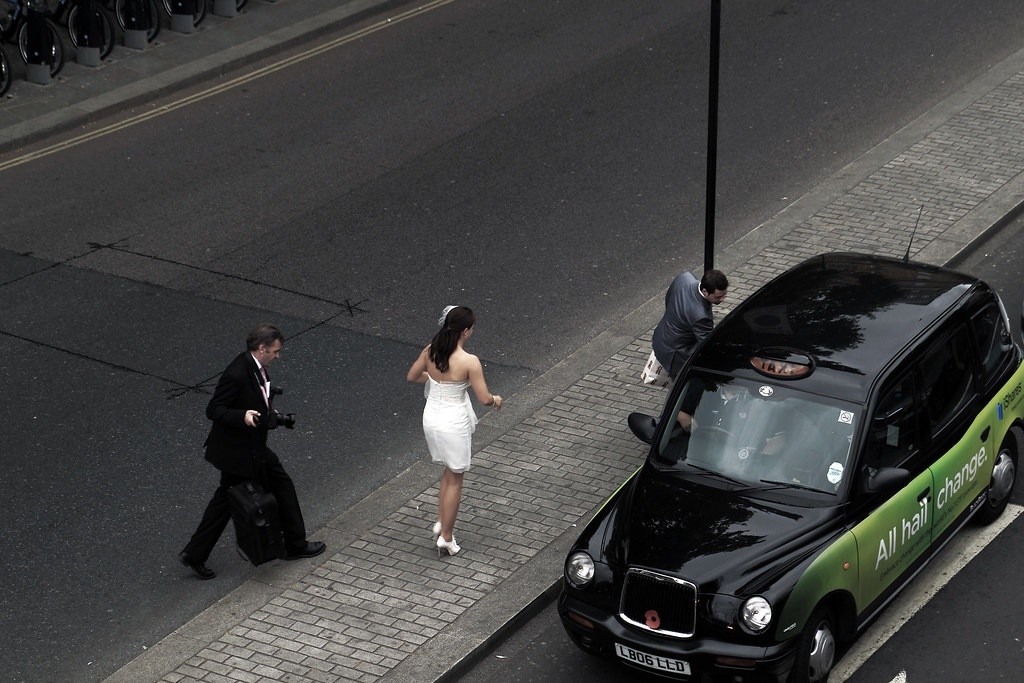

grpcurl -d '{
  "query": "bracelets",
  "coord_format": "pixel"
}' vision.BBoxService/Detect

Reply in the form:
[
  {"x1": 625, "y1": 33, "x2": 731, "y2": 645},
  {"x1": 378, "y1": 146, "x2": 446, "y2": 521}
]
[{"x1": 490, "y1": 396, "x2": 496, "y2": 406}]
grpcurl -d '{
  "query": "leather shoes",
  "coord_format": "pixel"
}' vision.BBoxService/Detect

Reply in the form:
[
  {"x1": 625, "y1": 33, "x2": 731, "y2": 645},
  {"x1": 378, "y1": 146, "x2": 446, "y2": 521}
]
[
  {"x1": 282, "y1": 542, "x2": 326, "y2": 561},
  {"x1": 178, "y1": 548, "x2": 216, "y2": 580}
]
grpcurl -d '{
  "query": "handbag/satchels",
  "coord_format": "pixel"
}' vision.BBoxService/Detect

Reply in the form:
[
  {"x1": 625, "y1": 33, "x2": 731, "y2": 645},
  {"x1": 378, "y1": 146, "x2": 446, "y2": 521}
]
[{"x1": 640, "y1": 348, "x2": 673, "y2": 389}]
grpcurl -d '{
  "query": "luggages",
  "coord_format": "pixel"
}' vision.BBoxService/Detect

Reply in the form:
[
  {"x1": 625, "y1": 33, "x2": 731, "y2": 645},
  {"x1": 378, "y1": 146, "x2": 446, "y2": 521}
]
[{"x1": 228, "y1": 480, "x2": 284, "y2": 567}]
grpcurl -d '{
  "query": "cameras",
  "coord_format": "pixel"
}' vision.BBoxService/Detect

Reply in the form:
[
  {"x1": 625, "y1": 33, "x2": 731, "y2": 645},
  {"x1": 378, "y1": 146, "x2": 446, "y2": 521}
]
[{"x1": 267, "y1": 409, "x2": 296, "y2": 431}]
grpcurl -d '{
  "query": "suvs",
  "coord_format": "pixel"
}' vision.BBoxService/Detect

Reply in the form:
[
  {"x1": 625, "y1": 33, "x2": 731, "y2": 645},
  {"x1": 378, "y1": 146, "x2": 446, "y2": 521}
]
[{"x1": 553, "y1": 248, "x2": 1024, "y2": 683}]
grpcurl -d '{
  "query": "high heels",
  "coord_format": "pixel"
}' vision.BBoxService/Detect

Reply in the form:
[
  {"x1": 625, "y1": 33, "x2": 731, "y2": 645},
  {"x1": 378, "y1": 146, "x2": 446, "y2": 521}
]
[
  {"x1": 433, "y1": 521, "x2": 442, "y2": 541},
  {"x1": 437, "y1": 535, "x2": 461, "y2": 557}
]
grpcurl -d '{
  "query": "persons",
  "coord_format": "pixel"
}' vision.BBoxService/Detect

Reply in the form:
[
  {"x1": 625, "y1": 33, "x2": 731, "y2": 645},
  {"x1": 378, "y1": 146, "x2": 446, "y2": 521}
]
[
  {"x1": 407, "y1": 304, "x2": 503, "y2": 557},
  {"x1": 652, "y1": 270, "x2": 729, "y2": 379},
  {"x1": 178, "y1": 324, "x2": 326, "y2": 579},
  {"x1": 677, "y1": 381, "x2": 787, "y2": 456}
]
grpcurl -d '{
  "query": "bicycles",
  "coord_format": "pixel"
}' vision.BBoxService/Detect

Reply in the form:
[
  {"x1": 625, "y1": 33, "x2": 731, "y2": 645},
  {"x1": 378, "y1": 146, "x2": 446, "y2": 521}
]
[
  {"x1": 0, "y1": 38, "x2": 15, "y2": 95},
  {"x1": 0, "y1": 0, "x2": 66, "y2": 79},
  {"x1": 24, "y1": 0, "x2": 117, "y2": 62},
  {"x1": 204, "y1": 0, "x2": 249, "y2": 16},
  {"x1": 153, "y1": 0, "x2": 208, "y2": 29},
  {"x1": 73, "y1": 0, "x2": 162, "y2": 43}
]
